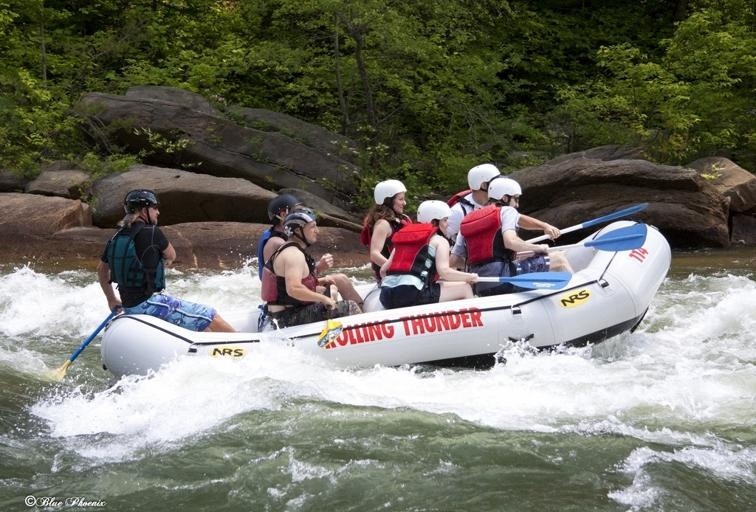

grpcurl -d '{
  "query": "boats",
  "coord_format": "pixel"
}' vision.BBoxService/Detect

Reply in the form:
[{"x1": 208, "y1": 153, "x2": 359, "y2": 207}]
[{"x1": 101, "y1": 219, "x2": 672, "y2": 380}]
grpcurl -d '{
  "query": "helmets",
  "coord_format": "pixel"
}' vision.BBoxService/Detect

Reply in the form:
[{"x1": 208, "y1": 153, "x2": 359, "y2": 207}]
[
  {"x1": 487, "y1": 178, "x2": 522, "y2": 200},
  {"x1": 282, "y1": 207, "x2": 316, "y2": 236},
  {"x1": 417, "y1": 201, "x2": 451, "y2": 224},
  {"x1": 375, "y1": 179, "x2": 407, "y2": 204},
  {"x1": 468, "y1": 164, "x2": 500, "y2": 190},
  {"x1": 267, "y1": 194, "x2": 302, "y2": 220},
  {"x1": 123, "y1": 189, "x2": 161, "y2": 206}
]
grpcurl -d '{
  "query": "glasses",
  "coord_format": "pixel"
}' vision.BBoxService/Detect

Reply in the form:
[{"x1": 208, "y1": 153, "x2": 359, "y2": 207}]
[{"x1": 511, "y1": 197, "x2": 519, "y2": 202}]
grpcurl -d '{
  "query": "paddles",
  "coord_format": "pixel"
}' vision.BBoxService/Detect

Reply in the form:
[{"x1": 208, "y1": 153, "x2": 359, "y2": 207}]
[
  {"x1": 525, "y1": 203, "x2": 649, "y2": 243},
  {"x1": 549, "y1": 225, "x2": 647, "y2": 251},
  {"x1": 477, "y1": 271, "x2": 571, "y2": 290},
  {"x1": 53, "y1": 306, "x2": 122, "y2": 381},
  {"x1": 317, "y1": 284, "x2": 344, "y2": 347}
]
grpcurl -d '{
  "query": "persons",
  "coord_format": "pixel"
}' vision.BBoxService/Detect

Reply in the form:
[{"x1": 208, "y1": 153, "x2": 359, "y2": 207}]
[
  {"x1": 97, "y1": 188, "x2": 235, "y2": 333},
  {"x1": 360, "y1": 179, "x2": 413, "y2": 288},
  {"x1": 378, "y1": 200, "x2": 478, "y2": 310},
  {"x1": 445, "y1": 163, "x2": 562, "y2": 253},
  {"x1": 258, "y1": 194, "x2": 364, "y2": 312},
  {"x1": 449, "y1": 177, "x2": 574, "y2": 278},
  {"x1": 257, "y1": 207, "x2": 362, "y2": 334}
]
